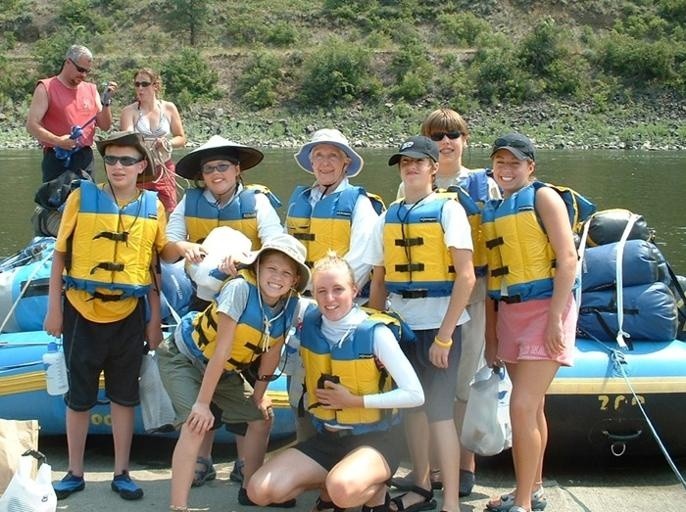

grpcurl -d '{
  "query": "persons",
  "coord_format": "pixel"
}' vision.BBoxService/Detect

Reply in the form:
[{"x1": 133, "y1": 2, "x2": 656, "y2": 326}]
[{"x1": 25, "y1": 46, "x2": 580, "y2": 512}]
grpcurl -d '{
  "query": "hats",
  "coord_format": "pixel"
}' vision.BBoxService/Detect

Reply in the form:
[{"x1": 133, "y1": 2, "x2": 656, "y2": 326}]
[
  {"x1": 240, "y1": 233, "x2": 312, "y2": 293},
  {"x1": 175, "y1": 135, "x2": 264, "y2": 179},
  {"x1": 294, "y1": 129, "x2": 363, "y2": 177},
  {"x1": 490, "y1": 131, "x2": 534, "y2": 159},
  {"x1": 95, "y1": 132, "x2": 154, "y2": 176},
  {"x1": 389, "y1": 136, "x2": 439, "y2": 166}
]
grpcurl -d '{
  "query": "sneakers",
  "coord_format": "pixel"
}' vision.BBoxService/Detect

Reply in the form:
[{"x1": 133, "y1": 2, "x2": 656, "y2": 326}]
[
  {"x1": 54, "y1": 471, "x2": 84, "y2": 499},
  {"x1": 112, "y1": 470, "x2": 143, "y2": 500},
  {"x1": 239, "y1": 487, "x2": 296, "y2": 507}
]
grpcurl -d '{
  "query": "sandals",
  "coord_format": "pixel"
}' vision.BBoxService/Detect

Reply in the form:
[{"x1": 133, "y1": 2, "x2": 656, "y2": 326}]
[
  {"x1": 230, "y1": 460, "x2": 246, "y2": 482},
  {"x1": 486, "y1": 486, "x2": 547, "y2": 512},
  {"x1": 312, "y1": 470, "x2": 475, "y2": 512},
  {"x1": 192, "y1": 457, "x2": 216, "y2": 487}
]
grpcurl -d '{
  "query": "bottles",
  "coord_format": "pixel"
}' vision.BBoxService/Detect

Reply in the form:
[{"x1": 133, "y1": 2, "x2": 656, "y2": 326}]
[
  {"x1": 280, "y1": 330, "x2": 302, "y2": 377},
  {"x1": 41, "y1": 341, "x2": 69, "y2": 397},
  {"x1": 492, "y1": 364, "x2": 514, "y2": 426}
]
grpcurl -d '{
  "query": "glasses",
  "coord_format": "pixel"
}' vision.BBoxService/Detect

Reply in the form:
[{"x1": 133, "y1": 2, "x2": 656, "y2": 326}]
[
  {"x1": 495, "y1": 138, "x2": 528, "y2": 147},
  {"x1": 431, "y1": 130, "x2": 463, "y2": 141},
  {"x1": 201, "y1": 165, "x2": 229, "y2": 173},
  {"x1": 68, "y1": 57, "x2": 91, "y2": 73},
  {"x1": 135, "y1": 82, "x2": 155, "y2": 87},
  {"x1": 103, "y1": 156, "x2": 142, "y2": 166}
]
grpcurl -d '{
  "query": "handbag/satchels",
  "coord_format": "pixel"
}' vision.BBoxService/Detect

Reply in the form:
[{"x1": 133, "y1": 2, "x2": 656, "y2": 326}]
[
  {"x1": 137, "y1": 343, "x2": 177, "y2": 434},
  {"x1": 32, "y1": 168, "x2": 93, "y2": 237},
  {"x1": 460, "y1": 364, "x2": 513, "y2": 456}
]
